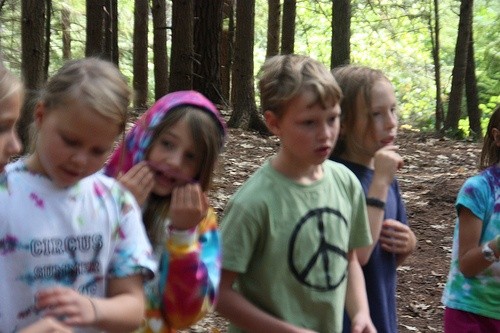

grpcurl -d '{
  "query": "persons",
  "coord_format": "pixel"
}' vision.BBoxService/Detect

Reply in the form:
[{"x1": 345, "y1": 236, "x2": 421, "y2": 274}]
[
  {"x1": 0, "y1": 62, "x2": 23, "y2": 177},
  {"x1": 0, "y1": 56, "x2": 158, "y2": 332},
  {"x1": 441, "y1": 102, "x2": 499, "y2": 333},
  {"x1": 328, "y1": 63, "x2": 417, "y2": 332},
  {"x1": 103, "y1": 90, "x2": 227, "y2": 333},
  {"x1": 214, "y1": 54, "x2": 377, "y2": 332}
]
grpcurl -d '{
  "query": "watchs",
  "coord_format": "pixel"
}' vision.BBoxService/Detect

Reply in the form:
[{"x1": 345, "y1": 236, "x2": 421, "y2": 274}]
[
  {"x1": 481, "y1": 241, "x2": 498, "y2": 264},
  {"x1": 366, "y1": 197, "x2": 386, "y2": 209}
]
